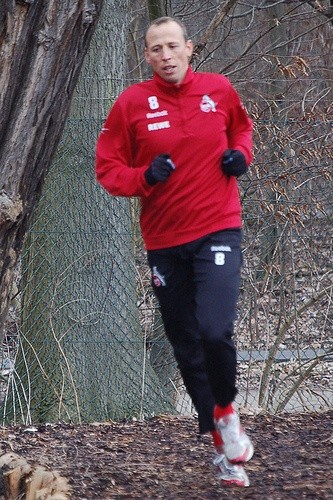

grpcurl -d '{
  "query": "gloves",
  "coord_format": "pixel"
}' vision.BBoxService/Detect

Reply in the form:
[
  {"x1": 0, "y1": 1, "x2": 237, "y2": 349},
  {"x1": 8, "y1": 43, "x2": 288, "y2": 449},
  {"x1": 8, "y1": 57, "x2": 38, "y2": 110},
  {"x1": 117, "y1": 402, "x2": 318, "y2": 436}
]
[
  {"x1": 145, "y1": 154, "x2": 173, "y2": 186},
  {"x1": 222, "y1": 149, "x2": 248, "y2": 176}
]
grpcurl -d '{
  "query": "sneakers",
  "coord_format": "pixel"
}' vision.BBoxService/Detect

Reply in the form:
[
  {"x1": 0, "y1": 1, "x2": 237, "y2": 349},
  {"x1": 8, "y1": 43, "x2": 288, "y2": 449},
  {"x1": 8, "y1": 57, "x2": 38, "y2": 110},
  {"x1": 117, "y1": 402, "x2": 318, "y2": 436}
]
[
  {"x1": 215, "y1": 409, "x2": 254, "y2": 464},
  {"x1": 213, "y1": 453, "x2": 250, "y2": 488}
]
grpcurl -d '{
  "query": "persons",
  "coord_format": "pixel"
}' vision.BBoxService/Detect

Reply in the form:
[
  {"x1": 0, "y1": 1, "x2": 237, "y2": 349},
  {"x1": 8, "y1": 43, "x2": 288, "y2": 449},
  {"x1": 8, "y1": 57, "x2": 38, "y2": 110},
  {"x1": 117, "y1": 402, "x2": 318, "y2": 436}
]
[{"x1": 93, "y1": 15, "x2": 257, "y2": 489}]
